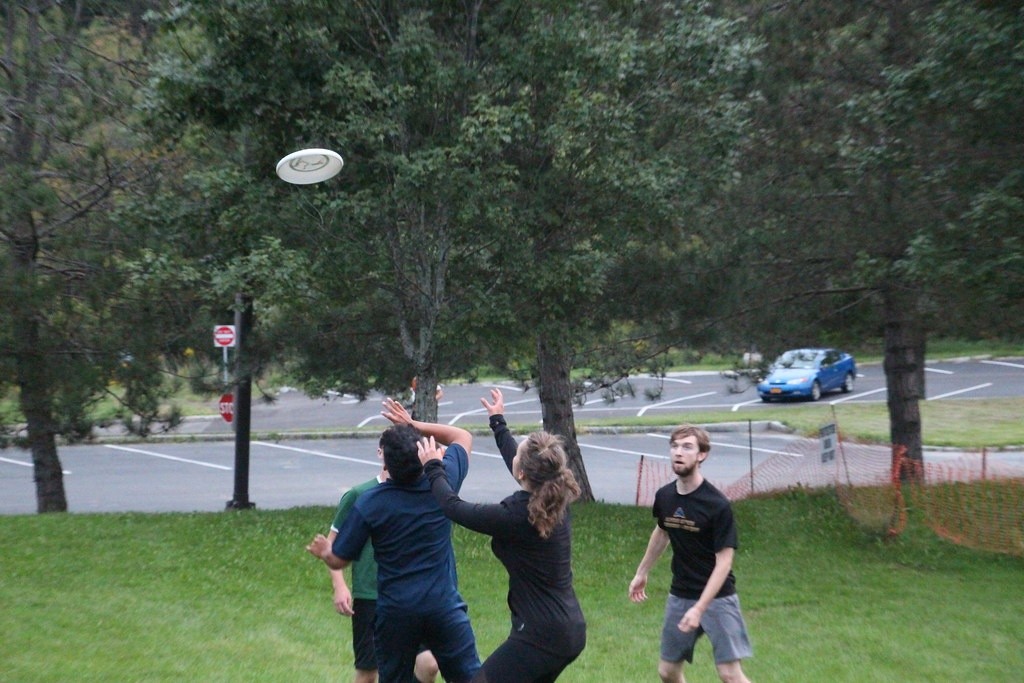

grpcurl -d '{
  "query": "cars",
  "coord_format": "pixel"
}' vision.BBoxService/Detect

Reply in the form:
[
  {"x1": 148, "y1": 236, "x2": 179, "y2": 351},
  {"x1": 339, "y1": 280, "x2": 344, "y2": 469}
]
[{"x1": 755, "y1": 346, "x2": 857, "y2": 403}]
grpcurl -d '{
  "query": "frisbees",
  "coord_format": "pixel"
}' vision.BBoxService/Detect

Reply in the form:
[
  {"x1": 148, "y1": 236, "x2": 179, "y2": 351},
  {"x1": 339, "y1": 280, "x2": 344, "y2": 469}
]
[{"x1": 274, "y1": 147, "x2": 345, "y2": 186}]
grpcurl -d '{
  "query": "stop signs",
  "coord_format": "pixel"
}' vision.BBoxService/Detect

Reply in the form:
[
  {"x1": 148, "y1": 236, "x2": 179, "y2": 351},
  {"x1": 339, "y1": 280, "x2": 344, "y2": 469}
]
[
  {"x1": 213, "y1": 325, "x2": 236, "y2": 347},
  {"x1": 220, "y1": 394, "x2": 235, "y2": 424}
]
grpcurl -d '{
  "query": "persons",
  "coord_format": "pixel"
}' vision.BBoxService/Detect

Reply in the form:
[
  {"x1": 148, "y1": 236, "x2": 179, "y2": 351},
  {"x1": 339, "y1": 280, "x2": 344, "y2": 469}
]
[
  {"x1": 304, "y1": 393, "x2": 484, "y2": 683},
  {"x1": 627, "y1": 425, "x2": 756, "y2": 683},
  {"x1": 326, "y1": 431, "x2": 439, "y2": 683},
  {"x1": 414, "y1": 385, "x2": 589, "y2": 683},
  {"x1": 405, "y1": 376, "x2": 442, "y2": 424}
]
